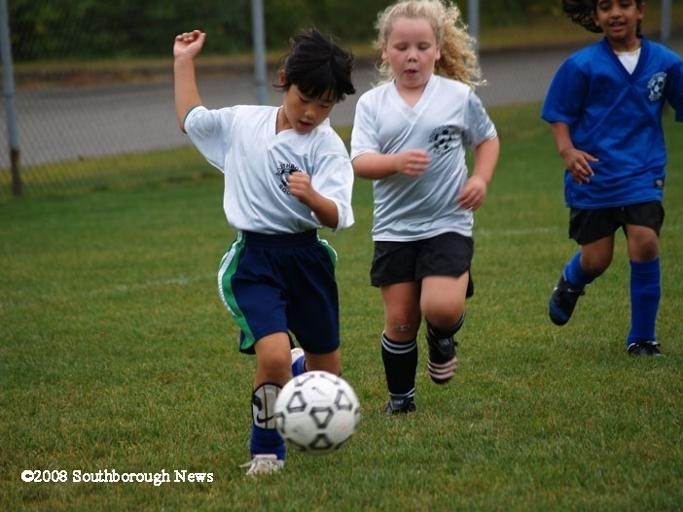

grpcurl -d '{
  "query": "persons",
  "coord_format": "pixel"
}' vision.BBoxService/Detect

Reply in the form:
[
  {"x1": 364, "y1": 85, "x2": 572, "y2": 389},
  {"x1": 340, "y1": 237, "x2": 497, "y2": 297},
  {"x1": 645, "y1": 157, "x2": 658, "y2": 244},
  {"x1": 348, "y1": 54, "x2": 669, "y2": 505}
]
[
  {"x1": 172, "y1": 27, "x2": 358, "y2": 478},
  {"x1": 539, "y1": 0, "x2": 683, "y2": 358},
  {"x1": 349, "y1": 0, "x2": 504, "y2": 414}
]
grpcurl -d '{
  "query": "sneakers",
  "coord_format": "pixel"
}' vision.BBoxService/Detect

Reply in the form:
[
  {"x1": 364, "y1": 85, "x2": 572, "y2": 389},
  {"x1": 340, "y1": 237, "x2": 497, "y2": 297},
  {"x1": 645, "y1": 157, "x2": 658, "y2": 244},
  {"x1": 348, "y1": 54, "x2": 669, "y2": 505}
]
[
  {"x1": 243, "y1": 452, "x2": 286, "y2": 482},
  {"x1": 291, "y1": 348, "x2": 306, "y2": 377},
  {"x1": 626, "y1": 342, "x2": 663, "y2": 358},
  {"x1": 548, "y1": 272, "x2": 586, "y2": 326},
  {"x1": 373, "y1": 396, "x2": 417, "y2": 419},
  {"x1": 428, "y1": 328, "x2": 458, "y2": 385}
]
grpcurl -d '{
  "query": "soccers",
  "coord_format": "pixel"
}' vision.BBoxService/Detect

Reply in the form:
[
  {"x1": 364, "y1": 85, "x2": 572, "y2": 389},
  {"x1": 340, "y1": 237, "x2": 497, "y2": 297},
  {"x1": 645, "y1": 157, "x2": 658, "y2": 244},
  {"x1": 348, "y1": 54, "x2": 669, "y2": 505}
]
[{"x1": 271, "y1": 370, "x2": 364, "y2": 455}]
